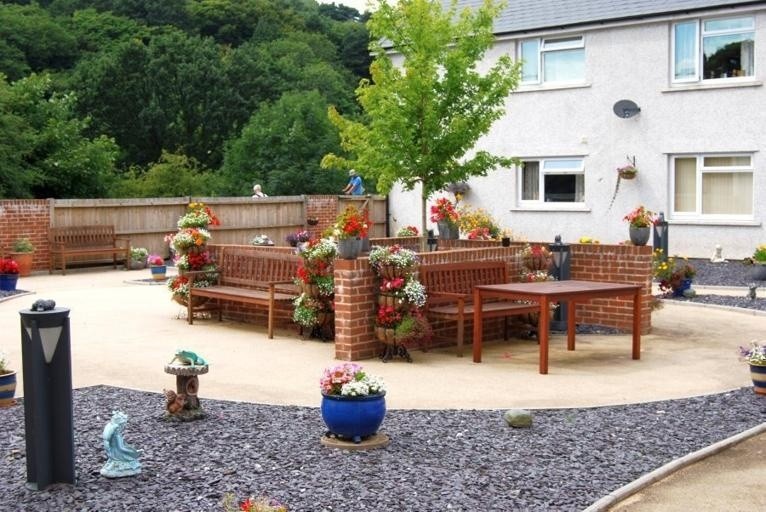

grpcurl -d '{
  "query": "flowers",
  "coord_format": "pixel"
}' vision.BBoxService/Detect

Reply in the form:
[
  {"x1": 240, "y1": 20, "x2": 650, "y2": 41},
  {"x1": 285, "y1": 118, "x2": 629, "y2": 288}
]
[
  {"x1": 167, "y1": 276, "x2": 213, "y2": 297},
  {"x1": 292, "y1": 293, "x2": 335, "y2": 328},
  {"x1": 147, "y1": 254, "x2": 164, "y2": 265},
  {"x1": 320, "y1": 363, "x2": 387, "y2": 396},
  {"x1": 173, "y1": 248, "x2": 218, "y2": 277},
  {"x1": 375, "y1": 306, "x2": 433, "y2": 349},
  {"x1": 170, "y1": 203, "x2": 220, "y2": 227},
  {"x1": 323, "y1": 204, "x2": 375, "y2": 240},
  {"x1": 164, "y1": 227, "x2": 213, "y2": 255},
  {"x1": 398, "y1": 226, "x2": 418, "y2": 237},
  {"x1": 430, "y1": 193, "x2": 472, "y2": 224},
  {"x1": 623, "y1": 206, "x2": 659, "y2": 228},
  {"x1": 460, "y1": 208, "x2": 502, "y2": 241},
  {"x1": 739, "y1": 339, "x2": 766, "y2": 366},
  {"x1": 502, "y1": 228, "x2": 512, "y2": 238},
  {"x1": 292, "y1": 258, "x2": 334, "y2": 296},
  {"x1": 444, "y1": 183, "x2": 471, "y2": 194},
  {"x1": 520, "y1": 271, "x2": 555, "y2": 281},
  {"x1": 437, "y1": 222, "x2": 459, "y2": 240},
  {"x1": 651, "y1": 248, "x2": 697, "y2": 292},
  {"x1": 515, "y1": 243, "x2": 553, "y2": 270},
  {"x1": 297, "y1": 231, "x2": 310, "y2": 242},
  {"x1": 0, "y1": 258, "x2": 19, "y2": 273},
  {"x1": 618, "y1": 166, "x2": 637, "y2": 174},
  {"x1": 379, "y1": 278, "x2": 429, "y2": 309},
  {"x1": 298, "y1": 238, "x2": 337, "y2": 271},
  {"x1": 285, "y1": 234, "x2": 299, "y2": 241}
]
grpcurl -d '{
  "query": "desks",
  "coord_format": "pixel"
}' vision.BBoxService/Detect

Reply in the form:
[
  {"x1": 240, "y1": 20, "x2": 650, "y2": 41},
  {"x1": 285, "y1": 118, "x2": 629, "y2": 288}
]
[{"x1": 474, "y1": 280, "x2": 643, "y2": 374}]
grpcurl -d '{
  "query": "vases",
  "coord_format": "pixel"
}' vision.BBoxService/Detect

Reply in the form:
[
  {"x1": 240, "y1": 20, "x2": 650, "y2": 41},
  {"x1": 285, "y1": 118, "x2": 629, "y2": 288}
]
[
  {"x1": 0, "y1": 273, "x2": 18, "y2": 291},
  {"x1": 368, "y1": 244, "x2": 421, "y2": 270},
  {"x1": 524, "y1": 252, "x2": 547, "y2": 270},
  {"x1": 502, "y1": 238, "x2": 510, "y2": 247},
  {"x1": 375, "y1": 328, "x2": 424, "y2": 345},
  {"x1": 321, "y1": 390, "x2": 386, "y2": 444},
  {"x1": 630, "y1": 226, "x2": 651, "y2": 245},
  {"x1": 318, "y1": 313, "x2": 334, "y2": 326},
  {"x1": 172, "y1": 295, "x2": 209, "y2": 307},
  {"x1": 301, "y1": 280, "x2": 320, "y2": 297},
  {"x1": 748, "y1": 362, "x2": 766, "y2": 394},
  {"x1": 620, "y1": 173, "x2": 635, "y2": 180},
  {"x1": 381, "y1": 266, "x2": 405, "y2": 279},
  {"x1": 673, "y1": 280, "x2": 692, "y2": 296},
  {"x1": 150, "y1": 265, "x2": 166, "y2": 280},
  {"x1": 290, "y1": 242, "x2": 297, "y2": 246},
  {"x1": 187, "y1": 247, "x2": 208, "y2": 256},
  {"x1": 454, "y1": 193, "x2": 464, "y2": 202},
  {"x1": 378, "y1": 292, "x2": 406, "y2": 311},
  {"x1": 179, "y1": 270, "x2": 204, "y2": 280},
  {"x1": 299, "y1": 241, "x2": 310, "y2": 251},
  {"x1": 338, "y1": 237, "x2": 363, "y2": 259}
]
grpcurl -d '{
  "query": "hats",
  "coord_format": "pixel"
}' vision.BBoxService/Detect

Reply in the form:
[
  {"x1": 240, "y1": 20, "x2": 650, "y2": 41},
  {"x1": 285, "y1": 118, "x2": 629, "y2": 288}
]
[{"x1": 349, "y1": 169, "x2": 356, "y2": 176}]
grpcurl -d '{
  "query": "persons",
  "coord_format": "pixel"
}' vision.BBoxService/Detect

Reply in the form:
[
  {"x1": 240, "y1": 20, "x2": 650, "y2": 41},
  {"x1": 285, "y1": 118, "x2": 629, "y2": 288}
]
[
  {"x1": 252, "y1": 184, "x2": 268, "y2": 198},
  {"x1": 343, "y1": 169, "x2": 365, "y2": 194}
]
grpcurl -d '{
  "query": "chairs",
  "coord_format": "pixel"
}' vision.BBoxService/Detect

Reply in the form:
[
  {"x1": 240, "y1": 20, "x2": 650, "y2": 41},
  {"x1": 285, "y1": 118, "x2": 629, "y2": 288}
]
[
  {"x1": 185, "y1": 248, "x2": 300, "y2": 339},
  {"x1": 419, "y1": 261, "x2": 541, "y2": 357},
  {"x1": 47, "y1": 225, "x2": 132, "y2": 276}
]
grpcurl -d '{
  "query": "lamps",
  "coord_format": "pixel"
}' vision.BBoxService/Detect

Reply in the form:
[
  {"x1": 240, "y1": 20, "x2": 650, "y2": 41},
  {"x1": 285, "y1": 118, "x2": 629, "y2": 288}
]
[{"x1": 613, "y1": 100, "x2": 641, "y2": 118}]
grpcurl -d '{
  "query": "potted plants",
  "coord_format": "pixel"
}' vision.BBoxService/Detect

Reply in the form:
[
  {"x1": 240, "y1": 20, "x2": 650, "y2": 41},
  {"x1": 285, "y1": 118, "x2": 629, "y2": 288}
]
[
  {"x1": 0, "y1": 359, "x2": 17, "y2": 405},
  {"x1": 6, "y1": 238, "x2": 36, "y2": 276},
  {"x1": 131, "y1": 248, "x2": 148, "y2": 269}
]
